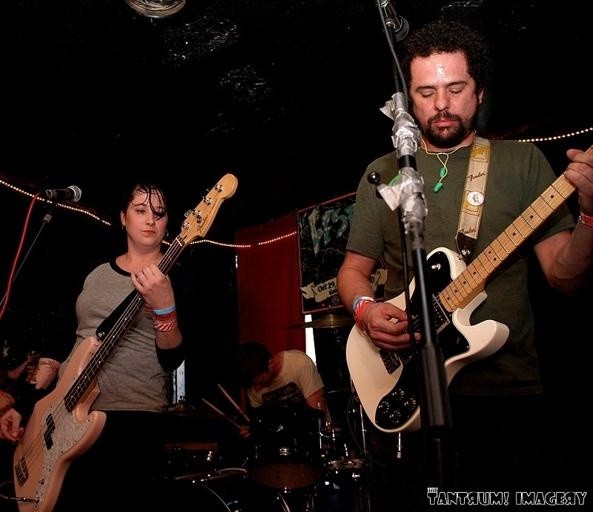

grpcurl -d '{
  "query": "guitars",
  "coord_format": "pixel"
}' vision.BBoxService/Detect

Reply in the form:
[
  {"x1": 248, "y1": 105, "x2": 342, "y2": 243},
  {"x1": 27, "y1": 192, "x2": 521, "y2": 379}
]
[
  {"x1": 346, "y1": 147, "x2": 591, "y2": 433},
  {"x1": 13, "y1": 174, "x2": 238, "y2": 512}
]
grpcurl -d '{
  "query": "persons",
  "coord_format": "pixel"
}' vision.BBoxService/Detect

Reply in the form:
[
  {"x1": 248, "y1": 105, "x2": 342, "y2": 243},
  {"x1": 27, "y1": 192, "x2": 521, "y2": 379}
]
[
  {"x1": 230, "y1": 339, "x2": 332, "y2": 439},
  {"x1": 0, "y1": 178, "x2": 195, "y2": 512},
  {"x1": 335, "y1": 17, "x2": 593, "y2": 512}
]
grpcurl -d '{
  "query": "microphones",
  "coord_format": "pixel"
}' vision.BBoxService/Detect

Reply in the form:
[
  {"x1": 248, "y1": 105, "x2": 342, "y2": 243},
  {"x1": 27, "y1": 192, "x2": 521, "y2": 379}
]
[
  {"x1": 45, "y1": 185, "x2": 82, "y2": 202},
  {"x1": 380, "y1": 0, "x2": 410, "y2": 41}
]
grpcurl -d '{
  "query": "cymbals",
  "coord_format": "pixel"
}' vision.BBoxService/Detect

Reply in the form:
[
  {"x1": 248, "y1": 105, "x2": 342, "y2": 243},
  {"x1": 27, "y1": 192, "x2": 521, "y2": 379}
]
[{"x1": 284, "y1": 314, "x2": 355, "y2": 329}]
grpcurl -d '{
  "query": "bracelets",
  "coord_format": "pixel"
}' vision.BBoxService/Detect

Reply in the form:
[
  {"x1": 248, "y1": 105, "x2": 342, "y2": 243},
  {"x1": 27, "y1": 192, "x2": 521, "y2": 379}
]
[
  {"x1": 150, "y1": 304, "x2": 178, "y2": 331},
  {"x1": 578, "y1": 211, "x2": 593, "y2": 228},
  {"x1": 352, "y1": 295, "x2": 373, "y2": 332}
]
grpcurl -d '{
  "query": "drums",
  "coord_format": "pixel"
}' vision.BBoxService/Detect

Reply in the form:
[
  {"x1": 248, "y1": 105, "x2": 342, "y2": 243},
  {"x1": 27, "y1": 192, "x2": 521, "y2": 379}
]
[
  {"x1": 247, "y1": 405, "x2": 325, "y2": 493},
  {"x1": 323, "y1": 426, "x2": 368, "y2": 472}
]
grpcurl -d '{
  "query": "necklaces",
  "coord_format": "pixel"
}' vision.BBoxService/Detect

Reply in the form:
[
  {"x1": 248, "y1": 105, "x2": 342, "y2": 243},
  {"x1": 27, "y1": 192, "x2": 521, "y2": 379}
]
[{"x1": 417, "y1": 129, "x2": 476, "y2": 192}]
